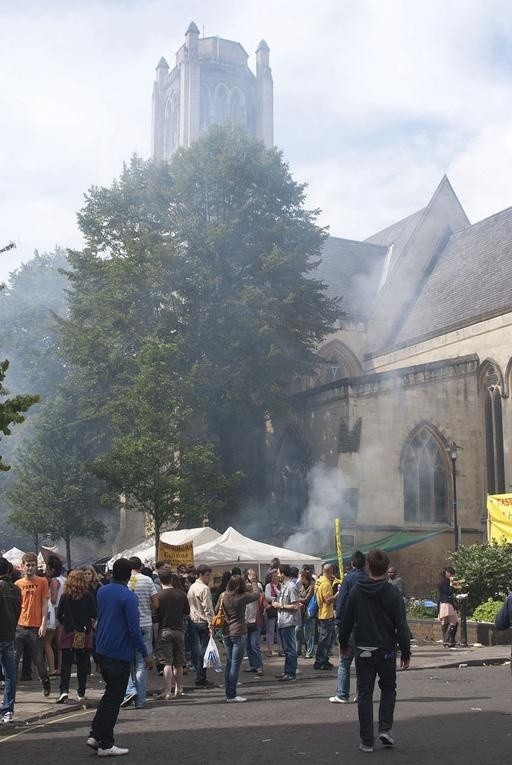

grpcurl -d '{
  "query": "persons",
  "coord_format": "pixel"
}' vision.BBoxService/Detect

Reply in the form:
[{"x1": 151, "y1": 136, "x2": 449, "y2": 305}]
[
  {"x1": 0, "y1": 550, "x2": 410, "y2": 759},
  {"x1": 437, "y1": 568, "x2": 458, "y2": 647},
  {"x1": 495, "y1": 596, "x2": 511, "y2": 630}
]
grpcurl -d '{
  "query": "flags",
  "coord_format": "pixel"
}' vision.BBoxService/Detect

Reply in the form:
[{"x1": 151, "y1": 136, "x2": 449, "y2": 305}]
[
  {"x1": 487, "y1": 494, "x2": 512, "y2": 546},
  {"x1": 159, "y1": 540, "x2": 193, "y2": 572}
]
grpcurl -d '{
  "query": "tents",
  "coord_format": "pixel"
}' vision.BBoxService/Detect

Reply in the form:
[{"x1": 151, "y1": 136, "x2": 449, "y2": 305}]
[{"x1": 105, "y1": 526, "x2": 322, "y2": 581}]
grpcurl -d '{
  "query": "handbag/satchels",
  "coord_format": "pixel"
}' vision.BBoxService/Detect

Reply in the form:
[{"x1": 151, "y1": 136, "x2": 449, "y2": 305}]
[
  {"x1": 72, "y1": 631, "x2": 87, "y2": 650},
  {"x1": 307, "y1": 594, "x2": 319, "y2": 620},
  {"x1": 267, "y1": 606, "x2": 281, "y2": 618},
  {"x1": 211, "y1": 614, "x2": 224, "y2": 626}
]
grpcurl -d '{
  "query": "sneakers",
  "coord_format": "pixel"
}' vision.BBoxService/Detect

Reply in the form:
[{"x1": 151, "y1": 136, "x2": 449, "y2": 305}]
[
  {"x1": 119, "y1": 661, "x2": 214, "y2": 709},
  {"x1": 379, "y1": 731, "x2": 394, "y2": 745},
  {"x1": 97, "y1": 745, "x2": 130, "y2": 756},
  {"x1": 87, "y1": 738, "x2": 101, "y2": 750},
  {"x1": 244, "y1": 646, "x2": 333, "y2": 682},
  {"x1": 358, "y1": 744, "x2": 373, "y2": 753},
  {"x1": 1, "y1": 712, "x2": 14, "y2": 723},
  {"x1": 18, "y1": 668, "x2": 87, "y2": 703},
  {"x1": 227, "y1": 696, "x2": 248, "y2": 703},
  {"x1": 329, "y1": 696, "x2": 349, "y2": 705}
]
